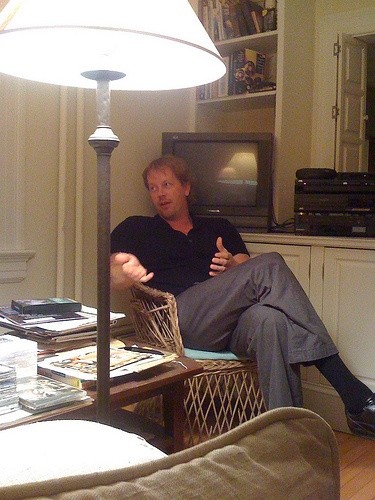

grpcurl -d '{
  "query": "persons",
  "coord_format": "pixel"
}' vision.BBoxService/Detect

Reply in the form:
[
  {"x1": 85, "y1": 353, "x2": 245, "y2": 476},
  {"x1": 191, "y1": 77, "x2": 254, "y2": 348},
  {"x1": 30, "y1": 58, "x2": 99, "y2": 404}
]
[{"x1": 109, "y1": 154, "x2": 375, "y2": 443}]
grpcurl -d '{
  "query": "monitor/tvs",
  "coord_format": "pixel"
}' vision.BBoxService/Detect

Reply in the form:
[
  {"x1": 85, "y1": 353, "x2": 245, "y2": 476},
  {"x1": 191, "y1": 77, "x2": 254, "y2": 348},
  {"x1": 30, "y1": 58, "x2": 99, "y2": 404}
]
[{"x1": 162, "y1": 131, "x2": 274, "y2": 232}]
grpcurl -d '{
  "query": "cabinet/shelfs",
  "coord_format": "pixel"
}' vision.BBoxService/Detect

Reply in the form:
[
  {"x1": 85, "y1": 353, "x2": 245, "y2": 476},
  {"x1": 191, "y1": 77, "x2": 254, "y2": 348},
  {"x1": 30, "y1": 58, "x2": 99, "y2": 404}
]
[
  {"x1": 239, "y1": 242, "x2": 375, "y2": 435},
  {"x1": 190, "y1": 0, "x2": 315, "y2": 232}
]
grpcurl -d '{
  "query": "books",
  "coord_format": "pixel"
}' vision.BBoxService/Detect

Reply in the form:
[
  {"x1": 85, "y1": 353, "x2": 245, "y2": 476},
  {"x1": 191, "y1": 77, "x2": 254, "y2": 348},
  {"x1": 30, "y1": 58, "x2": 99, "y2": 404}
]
[
  {"x1": 0, "y1": 297, "x2": 179, "y2": 428},
  {"x1": 197, "y1": 0, "x2": 278, "y2": 100}
]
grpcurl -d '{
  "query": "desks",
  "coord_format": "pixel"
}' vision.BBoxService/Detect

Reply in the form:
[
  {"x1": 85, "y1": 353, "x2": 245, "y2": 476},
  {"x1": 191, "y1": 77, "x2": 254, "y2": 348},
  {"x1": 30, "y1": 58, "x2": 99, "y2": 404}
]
[{"x1": 0, "y1": 339, "x2": 203, "y2": 454}]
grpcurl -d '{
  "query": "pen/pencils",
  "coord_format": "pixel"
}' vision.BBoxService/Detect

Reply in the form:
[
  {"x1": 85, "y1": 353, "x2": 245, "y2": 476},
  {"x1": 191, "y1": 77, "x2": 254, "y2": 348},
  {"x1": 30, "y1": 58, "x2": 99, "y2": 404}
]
[{"x1": 123, "y1": 347, "x2": 165, "y2": 355}]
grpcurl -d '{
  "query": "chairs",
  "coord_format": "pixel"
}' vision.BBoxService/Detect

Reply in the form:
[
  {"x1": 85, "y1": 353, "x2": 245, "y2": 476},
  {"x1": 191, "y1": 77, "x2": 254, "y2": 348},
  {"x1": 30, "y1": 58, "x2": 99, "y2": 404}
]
[{"x1": 131, "y1": 281, "x2": 268, "y2": 452}]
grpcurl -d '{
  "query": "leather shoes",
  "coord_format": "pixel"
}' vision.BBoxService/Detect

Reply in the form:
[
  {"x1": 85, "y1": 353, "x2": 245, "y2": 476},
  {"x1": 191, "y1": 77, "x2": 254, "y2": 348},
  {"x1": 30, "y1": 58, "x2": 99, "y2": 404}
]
[{"x1": 344, "y1": 393, "x2": 375, "y2": 442}]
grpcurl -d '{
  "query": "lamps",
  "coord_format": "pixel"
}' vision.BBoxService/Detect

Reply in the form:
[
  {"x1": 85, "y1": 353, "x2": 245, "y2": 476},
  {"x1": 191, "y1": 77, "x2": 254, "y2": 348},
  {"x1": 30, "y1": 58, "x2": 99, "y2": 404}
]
[
  {"x1": 216, "y1": 152, "x2": 259, "y2": 186},
  {"x1": 0, "y1": 2, "x2": 228, "y2": 427}
]
